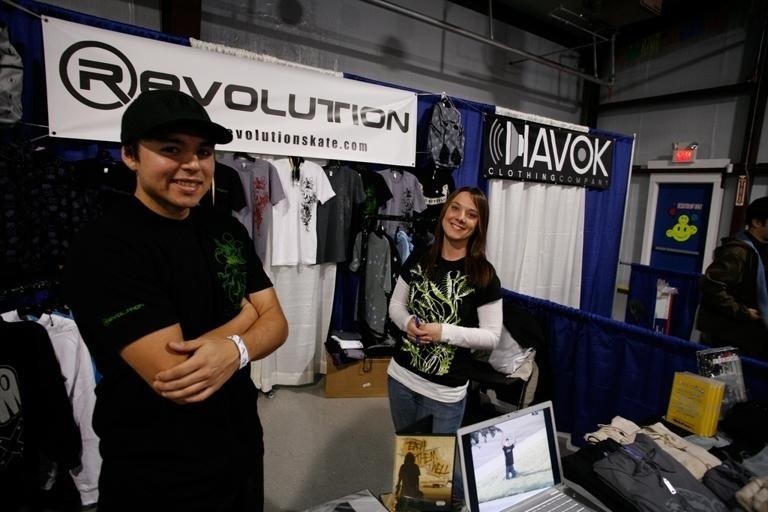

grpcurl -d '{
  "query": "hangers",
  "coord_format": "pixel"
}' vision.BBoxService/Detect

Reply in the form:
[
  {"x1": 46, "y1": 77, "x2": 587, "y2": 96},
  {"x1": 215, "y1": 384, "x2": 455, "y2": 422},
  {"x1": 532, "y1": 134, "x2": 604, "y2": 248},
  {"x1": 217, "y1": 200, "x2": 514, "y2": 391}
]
[
  {"x1": 17, "y1": 278, "x2": 70, "y2": 320},
  {"x1": 230, "y1": 153, "x2": 406, "y2": 178},
  {"x1": 365, "y1": 215, "x2": 430, "y2": 240}
]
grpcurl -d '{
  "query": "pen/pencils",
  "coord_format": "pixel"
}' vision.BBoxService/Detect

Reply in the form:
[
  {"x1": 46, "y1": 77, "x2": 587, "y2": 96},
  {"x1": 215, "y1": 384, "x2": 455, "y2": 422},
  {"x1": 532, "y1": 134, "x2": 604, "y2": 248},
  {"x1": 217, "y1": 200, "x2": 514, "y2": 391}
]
[{"x1": 416, "y1": 317, "x2": 420, "y2": 346}]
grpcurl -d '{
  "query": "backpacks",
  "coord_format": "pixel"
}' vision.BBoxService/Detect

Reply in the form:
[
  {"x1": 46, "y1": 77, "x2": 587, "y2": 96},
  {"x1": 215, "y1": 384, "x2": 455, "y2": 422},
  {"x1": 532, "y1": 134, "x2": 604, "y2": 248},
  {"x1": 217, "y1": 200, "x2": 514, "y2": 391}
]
[
  {"x1": 1, "y1": 24, "x2": 26, "y2": 123},
  {"x1": 426, "y1": 91, "x2": 467, "y2": 170}
]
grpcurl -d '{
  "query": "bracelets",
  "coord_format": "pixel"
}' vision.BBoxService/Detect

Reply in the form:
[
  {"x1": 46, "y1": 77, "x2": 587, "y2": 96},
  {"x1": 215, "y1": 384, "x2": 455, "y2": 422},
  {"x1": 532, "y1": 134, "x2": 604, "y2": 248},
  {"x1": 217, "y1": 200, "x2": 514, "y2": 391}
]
[{"x1": 226, "y1": 335, "x2": 249, "y2": 370}]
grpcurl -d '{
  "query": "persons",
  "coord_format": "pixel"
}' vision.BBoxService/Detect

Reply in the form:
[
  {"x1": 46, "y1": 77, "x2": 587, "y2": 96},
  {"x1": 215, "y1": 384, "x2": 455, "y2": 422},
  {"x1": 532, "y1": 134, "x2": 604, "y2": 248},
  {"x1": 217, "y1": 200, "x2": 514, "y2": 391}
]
[
  {"x1": 503, "y1": 438, "x2": 517, "y2": 480},
  {"x1": 58, "y1": 88, "x2": 289, "y2": 511},
  {"x1": 388, "y1": 187, "x2": 504, "y2": 436},
  {"x1": 395, "y1": 452, "x2": 422, "y2": 499},
  {"x1": 694, "y1": 196, "x2": 768, "y2": 361}
]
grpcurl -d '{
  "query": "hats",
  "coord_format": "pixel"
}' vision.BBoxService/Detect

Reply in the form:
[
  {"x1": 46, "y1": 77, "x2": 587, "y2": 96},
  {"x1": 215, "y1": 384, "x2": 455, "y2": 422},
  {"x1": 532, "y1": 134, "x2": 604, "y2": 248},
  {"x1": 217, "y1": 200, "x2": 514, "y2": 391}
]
[{"x1": 121, "y1": 90, "x2": 233, "y2": 144}]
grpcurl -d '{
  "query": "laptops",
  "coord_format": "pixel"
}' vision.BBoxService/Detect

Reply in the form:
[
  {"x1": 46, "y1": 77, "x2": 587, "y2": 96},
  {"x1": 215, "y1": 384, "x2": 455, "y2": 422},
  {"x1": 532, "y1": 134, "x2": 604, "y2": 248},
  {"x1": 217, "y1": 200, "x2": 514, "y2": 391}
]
[{"x1": 456, "y1": 400, "x2": 596, "y2": 512}]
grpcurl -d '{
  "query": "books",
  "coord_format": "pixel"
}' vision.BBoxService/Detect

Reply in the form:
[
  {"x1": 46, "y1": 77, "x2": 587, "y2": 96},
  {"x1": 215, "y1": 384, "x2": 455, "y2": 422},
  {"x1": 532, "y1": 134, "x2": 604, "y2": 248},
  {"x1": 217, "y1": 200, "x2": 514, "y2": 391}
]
[{"x1": 666, "y1": 371, "x2": 726, "y2": 437}]
